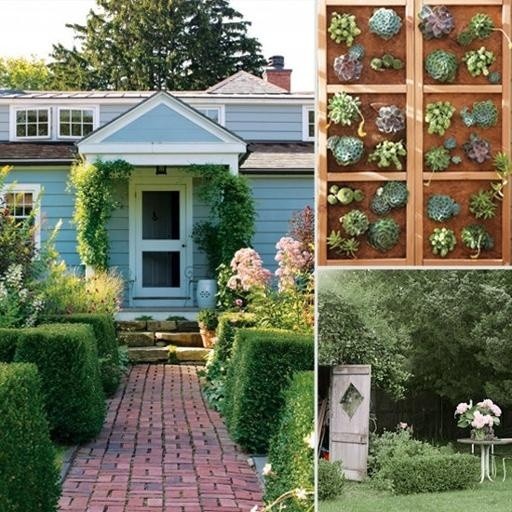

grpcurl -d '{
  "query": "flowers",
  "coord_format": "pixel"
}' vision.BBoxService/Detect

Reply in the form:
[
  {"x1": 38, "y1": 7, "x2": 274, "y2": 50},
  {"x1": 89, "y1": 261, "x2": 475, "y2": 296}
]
[{"x1": 454, "y1": 399, "x2": 502, "y2": 440}]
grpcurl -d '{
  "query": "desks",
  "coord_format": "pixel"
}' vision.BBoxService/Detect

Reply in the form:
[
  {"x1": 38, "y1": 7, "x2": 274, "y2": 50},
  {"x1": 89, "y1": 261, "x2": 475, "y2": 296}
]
[{"x1": 457, "y1": 438, "x2": 512, "y2": 484}]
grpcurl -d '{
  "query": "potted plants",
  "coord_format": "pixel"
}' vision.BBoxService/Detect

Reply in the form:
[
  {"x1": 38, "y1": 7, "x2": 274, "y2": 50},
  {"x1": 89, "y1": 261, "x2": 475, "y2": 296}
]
[{"x1": 197, "y1": 308, "x2": 218, "y2": 348}]
